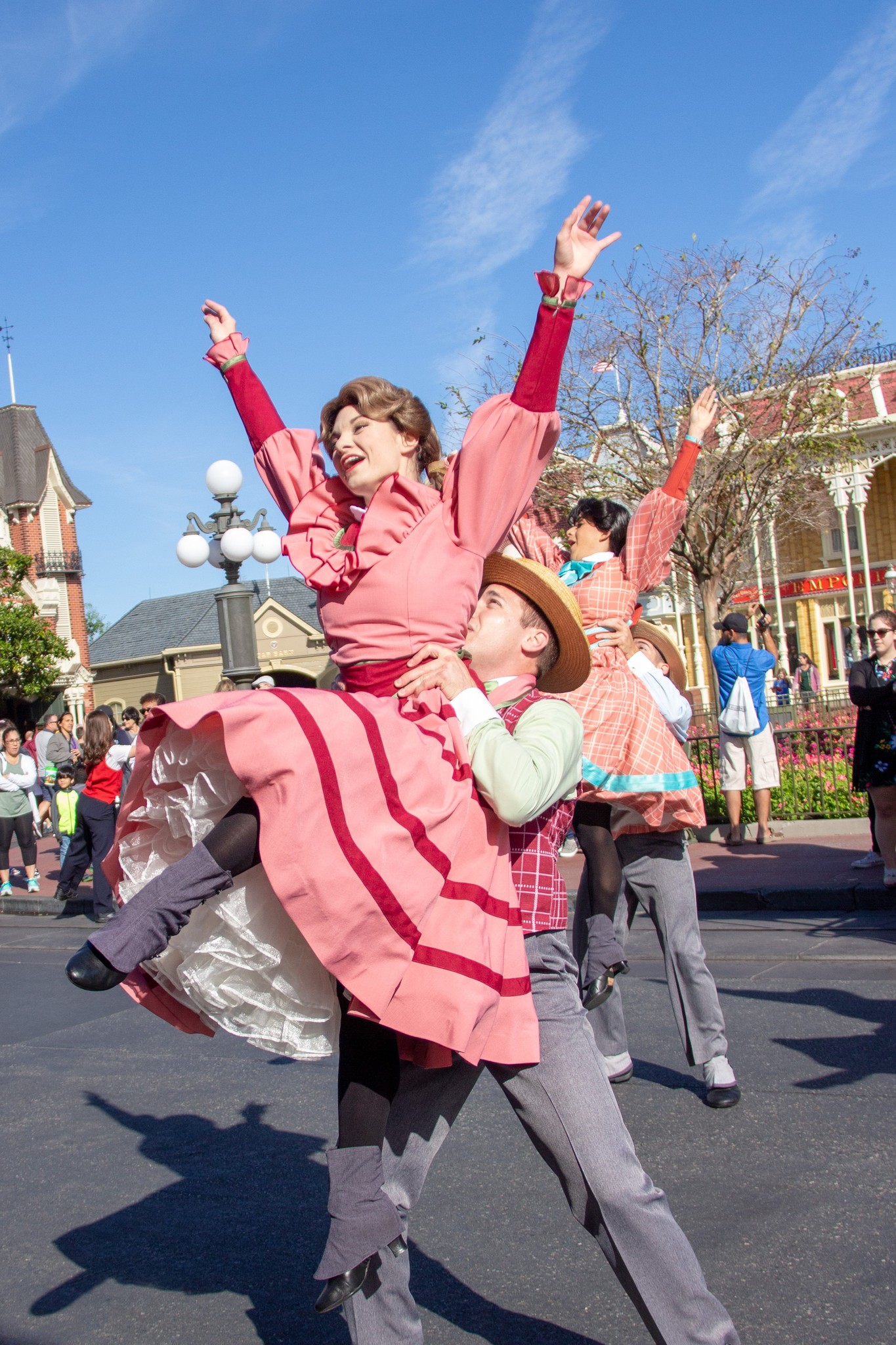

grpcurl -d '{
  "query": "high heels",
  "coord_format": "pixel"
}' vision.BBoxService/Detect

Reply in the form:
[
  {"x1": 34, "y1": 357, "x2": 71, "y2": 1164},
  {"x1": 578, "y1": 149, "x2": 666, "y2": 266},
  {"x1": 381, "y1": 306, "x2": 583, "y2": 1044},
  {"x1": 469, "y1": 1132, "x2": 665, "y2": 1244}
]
[
  {"x1": 581, "y1": 961, "x2": 630, "y2": 1010},
  {"x1": 63, "y1": 940, "x2": 128, "y2": 992},
  {"x1": 314, "y1": 1234, "x2": 408, "y2": 1312}
]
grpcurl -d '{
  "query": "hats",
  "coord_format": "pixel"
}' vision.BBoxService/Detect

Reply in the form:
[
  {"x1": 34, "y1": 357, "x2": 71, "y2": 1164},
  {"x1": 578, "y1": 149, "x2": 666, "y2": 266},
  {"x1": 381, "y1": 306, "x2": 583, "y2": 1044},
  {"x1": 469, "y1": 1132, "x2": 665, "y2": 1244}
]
[
  {"x1": 714, "y1": 612, "x2": 748, "y2": 633},
  {"x1": 251, "y1": 676, "x2": 274, "y2": 686},
  {"x1": 482, "y1": 553, "x2": 592, "y2": 694},
  {"x1": 95, "y1": 704, "x2": 113, "y2": 716},
  {"x1": 631, "y1": 619, "x2": 687, "y2": 695}
]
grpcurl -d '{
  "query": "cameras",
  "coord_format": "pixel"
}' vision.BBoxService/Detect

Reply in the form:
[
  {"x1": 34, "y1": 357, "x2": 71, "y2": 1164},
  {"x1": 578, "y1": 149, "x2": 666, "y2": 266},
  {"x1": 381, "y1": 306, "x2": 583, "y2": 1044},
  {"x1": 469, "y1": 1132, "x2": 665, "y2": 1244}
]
[{"x1": 756, "y1": 604, "x2": 770, "y2": 626}]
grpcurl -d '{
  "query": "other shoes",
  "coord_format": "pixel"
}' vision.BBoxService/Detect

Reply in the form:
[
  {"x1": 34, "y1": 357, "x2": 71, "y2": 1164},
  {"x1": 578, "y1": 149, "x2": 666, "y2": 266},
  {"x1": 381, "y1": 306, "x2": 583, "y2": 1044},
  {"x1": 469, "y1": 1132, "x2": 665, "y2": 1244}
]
[{"x1": 884, "y1": 868, "x2": 896, "y2": 887}]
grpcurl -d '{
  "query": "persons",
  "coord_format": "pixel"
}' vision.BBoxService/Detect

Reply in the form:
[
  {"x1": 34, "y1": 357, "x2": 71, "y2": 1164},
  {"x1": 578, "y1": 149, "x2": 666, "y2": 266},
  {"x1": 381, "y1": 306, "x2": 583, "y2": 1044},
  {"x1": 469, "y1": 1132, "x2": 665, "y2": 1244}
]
[
  {"x1": 51, "y1": 765, "x2": 93, "y2": 882},
  {"x1": 53, "y1": 710, "x2": 137, "y2": 922},
  {"x1": 216, "y1": 679, "x2": 236, "y2": 692},
  {"x1": 46, "y1": 712, "x2": 84, "y2": 792},
  {"x1": 32, "y1": 713, "x2": 59, "y2": 838},
  {"x1": 579, "y1": 620, "x2": 740, "y2": 1109},
  {"x1": 347, "y1": 552, "x2": 741, "y2": 1345},
  {"x1": 791, "y1": 652, "x2": 821, "y2": 711},
  {"x1": 771, "y1": 667, "x2": 792, "y2": 705},
  {"x1": 64, "y1": 195, "x2": 622, "y2": 1314},
  {"x1": 506, "y1": 385, "x2": 719, "y2": 1006},
  {"x1": 122, "y1": 706, "x2": 142, "y2": 743},
  {"x1": 96, "y1": 704, "x2": 132, "y2": 800},
  {"x1": 848, "y1": 610, "x2": 896, "y2": 884},
  {"x1": 23, "y1": 730, "x2": 51, "y2": 830},
  {"x1": 252, "y1": 676, "x2": 275, "y2": 690},
  {"x1": 129, "y1": 692, "x2": 167, "y2": 771},
  {"x1": 76, "y1": 726, "x2": 84, "y2": 749},
  {"x1": 0, "y1": 718, "x2": 41, "y2": 880},
  {"x1": 711, "y1": 601, "x2": 785, "y2": 845},
  {"x1": 0, "y1": 727, "x2": 40, "y2": 896}
]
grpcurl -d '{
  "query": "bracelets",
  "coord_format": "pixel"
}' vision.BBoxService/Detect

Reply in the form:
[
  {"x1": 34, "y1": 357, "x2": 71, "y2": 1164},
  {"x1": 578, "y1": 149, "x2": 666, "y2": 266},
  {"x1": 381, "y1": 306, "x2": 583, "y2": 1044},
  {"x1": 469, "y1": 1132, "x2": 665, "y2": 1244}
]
[{"x1": 764, "y1": 633, "x2": 768, "y2": 636}]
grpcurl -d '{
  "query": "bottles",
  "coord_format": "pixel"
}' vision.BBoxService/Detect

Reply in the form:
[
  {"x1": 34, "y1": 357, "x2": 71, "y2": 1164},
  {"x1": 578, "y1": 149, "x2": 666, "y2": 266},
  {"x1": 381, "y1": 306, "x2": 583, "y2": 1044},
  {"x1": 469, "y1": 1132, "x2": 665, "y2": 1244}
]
[{"x1": 44, "y1": 761, "x2": 58, "y2": 787}]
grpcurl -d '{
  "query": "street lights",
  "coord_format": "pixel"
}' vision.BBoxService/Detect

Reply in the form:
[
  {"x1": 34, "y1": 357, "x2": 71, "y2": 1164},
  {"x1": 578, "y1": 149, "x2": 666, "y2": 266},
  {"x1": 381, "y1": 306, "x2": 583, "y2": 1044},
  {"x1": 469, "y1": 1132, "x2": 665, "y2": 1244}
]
[{"x1": 175, "y1": 460, "x2": 283, "y2": 690}]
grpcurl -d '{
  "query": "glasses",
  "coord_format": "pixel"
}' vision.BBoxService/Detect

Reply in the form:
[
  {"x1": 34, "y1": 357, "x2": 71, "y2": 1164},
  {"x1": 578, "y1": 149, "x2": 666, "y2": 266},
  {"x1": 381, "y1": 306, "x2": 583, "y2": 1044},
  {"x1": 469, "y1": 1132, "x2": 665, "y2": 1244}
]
[
  {"x1": 139, "y1": 709, "x2": 150, "y2": 714},
  {"x1": 4, "y1": 738, "x2": 22, "y2": 744},
  {"x1": 866, "y1": 629, "x2": 893, "y2": 638},
  {"x1": 255, "y1": 684, "x2": 266, "y2": 689},
  {"x1": 122, "y1": 717, "x2": 132, "y2": 721}
]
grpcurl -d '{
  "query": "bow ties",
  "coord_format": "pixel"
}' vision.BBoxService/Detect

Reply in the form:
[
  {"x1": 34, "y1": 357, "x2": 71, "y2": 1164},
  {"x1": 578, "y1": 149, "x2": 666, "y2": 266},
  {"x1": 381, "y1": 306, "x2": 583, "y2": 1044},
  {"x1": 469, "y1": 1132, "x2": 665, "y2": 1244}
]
[{"x1": 483, "y1": 680, "x2": 499, "y2": 694}]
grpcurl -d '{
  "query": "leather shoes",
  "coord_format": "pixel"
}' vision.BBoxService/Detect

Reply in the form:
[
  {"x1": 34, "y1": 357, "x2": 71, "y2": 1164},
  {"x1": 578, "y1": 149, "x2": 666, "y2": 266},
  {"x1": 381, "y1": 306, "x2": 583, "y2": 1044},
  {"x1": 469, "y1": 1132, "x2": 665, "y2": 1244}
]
[
  {"x1": 608, "y1": 1070, "x2": 632, "y2": 1082},
  {"x1": 708, "y1": 1085, "x2": 741, "y2": 1108}
]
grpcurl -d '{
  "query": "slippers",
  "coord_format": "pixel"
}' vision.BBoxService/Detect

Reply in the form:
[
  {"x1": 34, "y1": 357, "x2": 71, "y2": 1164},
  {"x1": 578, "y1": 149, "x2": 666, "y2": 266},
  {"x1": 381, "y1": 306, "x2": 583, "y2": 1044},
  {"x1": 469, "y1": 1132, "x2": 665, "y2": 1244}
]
[
  {"x1": 756, "y1": 827, "x2": 784, "y2": 844},
  {"x1": 726, "y1": 832, "x2": 743, "y2": 846}
]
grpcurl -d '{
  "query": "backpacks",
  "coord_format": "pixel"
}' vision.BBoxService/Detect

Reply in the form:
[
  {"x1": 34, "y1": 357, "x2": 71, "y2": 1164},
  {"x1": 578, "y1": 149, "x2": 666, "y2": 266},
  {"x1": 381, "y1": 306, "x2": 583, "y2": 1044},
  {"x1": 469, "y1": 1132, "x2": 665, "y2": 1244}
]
[{"x1": 717, "y1": 648, "x2": 761, "y2": 736}]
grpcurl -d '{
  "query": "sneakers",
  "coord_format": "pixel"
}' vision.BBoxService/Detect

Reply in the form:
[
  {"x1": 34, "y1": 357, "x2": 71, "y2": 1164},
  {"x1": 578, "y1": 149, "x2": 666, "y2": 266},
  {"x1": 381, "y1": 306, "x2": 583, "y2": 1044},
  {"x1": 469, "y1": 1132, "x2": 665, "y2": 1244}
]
[
  {"x1": 33, "y1": 821, "x2": 43, "y2": 838},
  {"x1": 851, "y1": 850, "x2": 885, "y2": 868},
  {"x1": 1, "y1": 861, "x2": 94, "y2": 901},
  {"x1": 95, "y1": 912, "x2": 117, "y2": 922},
  {"x1": 43, "y1": 824, "x2": 53, "y2": 835},
  {"x1": 557, "y1": 838, "x2": 577, "y2": 858}
]
